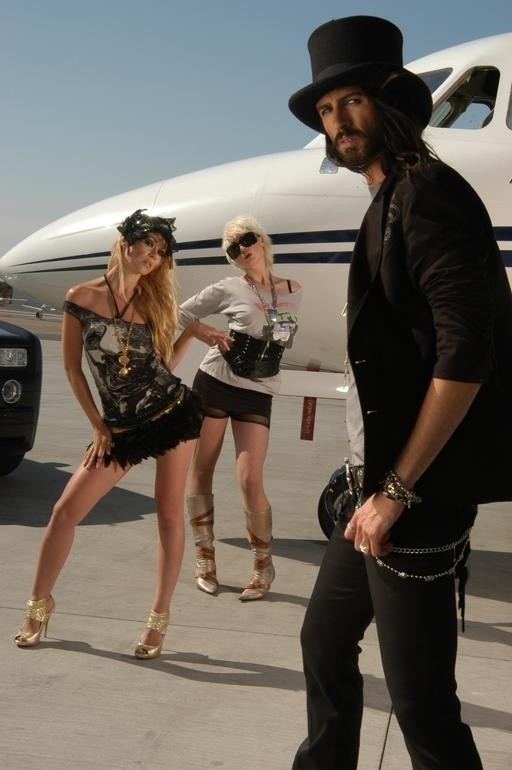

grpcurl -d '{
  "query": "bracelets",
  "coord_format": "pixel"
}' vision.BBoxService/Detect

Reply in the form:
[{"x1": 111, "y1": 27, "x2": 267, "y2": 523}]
[{"x1": 380, "y1": 468, "x2": 422, "y2": 514}]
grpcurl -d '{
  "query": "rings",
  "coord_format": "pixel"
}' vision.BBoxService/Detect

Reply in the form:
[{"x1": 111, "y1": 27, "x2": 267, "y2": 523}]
[
  {"x1": 359, "y1": 544, "x2": 370, "y2": 555},
  {"x1": 106, "y1": 442, "x2": 114, "y2": 448},
  {"x1": 96, "y1": 456, "x2": 104, "y2": 463},
  {"x1": 345, "y1": 523, "x2": 356, "y2": 532}
]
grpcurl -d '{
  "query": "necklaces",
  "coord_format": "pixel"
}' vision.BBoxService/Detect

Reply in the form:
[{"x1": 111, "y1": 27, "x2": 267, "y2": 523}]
[
  {"x1": 100, "y1": 272, "x2": 139, "y2": 326},
  {"x1": 103, "y1": 277, "x2": 138, "y2": 379},
  {"x1": 247, "y1": 268, "x2": 277, "y2": 346}
]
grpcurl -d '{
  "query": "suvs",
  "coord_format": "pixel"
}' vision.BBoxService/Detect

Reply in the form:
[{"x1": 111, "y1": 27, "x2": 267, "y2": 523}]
[{"x1": 2, "y1": 280, "x2": 43, "y2": 478}]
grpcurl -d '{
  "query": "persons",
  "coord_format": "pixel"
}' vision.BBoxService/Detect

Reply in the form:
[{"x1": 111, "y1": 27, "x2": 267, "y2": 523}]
[
  {"x1": 13, "y1": 206, "x2": 204, "y2": 662},
  {"x1": 175, "y1": 210, "x2": 303, "y2": 606},
  {"x1": 285, "y1": 16, "x2": 511, "y2": 770}
]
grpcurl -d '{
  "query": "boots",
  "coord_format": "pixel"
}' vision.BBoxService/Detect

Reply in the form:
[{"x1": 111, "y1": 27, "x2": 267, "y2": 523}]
[
  {"x1": 185, "y1": 494, "x2": 219, "y2": 594},
  {"x1": 238, "y1": 506, "x2": 275, "y2": 601}
]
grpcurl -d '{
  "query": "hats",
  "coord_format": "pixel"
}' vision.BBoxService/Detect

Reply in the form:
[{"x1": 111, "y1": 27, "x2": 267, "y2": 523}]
[
  {"x1": 289, "y1": 14, "x2": 434, "y2": 133},
  {"x1": 138, "y1": 217, "x2": 172, "y2": 243}
]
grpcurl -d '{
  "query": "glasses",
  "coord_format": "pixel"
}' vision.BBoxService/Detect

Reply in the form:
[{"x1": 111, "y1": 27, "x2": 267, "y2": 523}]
[{"x1": 226, "y1": 232, "x2": 258, "y2": 260}]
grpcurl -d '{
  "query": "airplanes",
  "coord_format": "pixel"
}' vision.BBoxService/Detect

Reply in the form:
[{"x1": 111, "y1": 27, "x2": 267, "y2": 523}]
[{"x1": 1, "y1": 31, "x2": 510, "y2": 539}]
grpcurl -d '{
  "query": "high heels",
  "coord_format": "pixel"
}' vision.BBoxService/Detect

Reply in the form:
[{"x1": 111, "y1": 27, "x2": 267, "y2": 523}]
[
  {"x1": 14, "y1": 593, "x2": 56, "y2": 647},
  {"x1": 135, "y1": 608, "x2": 170, "y2": 660}
]
